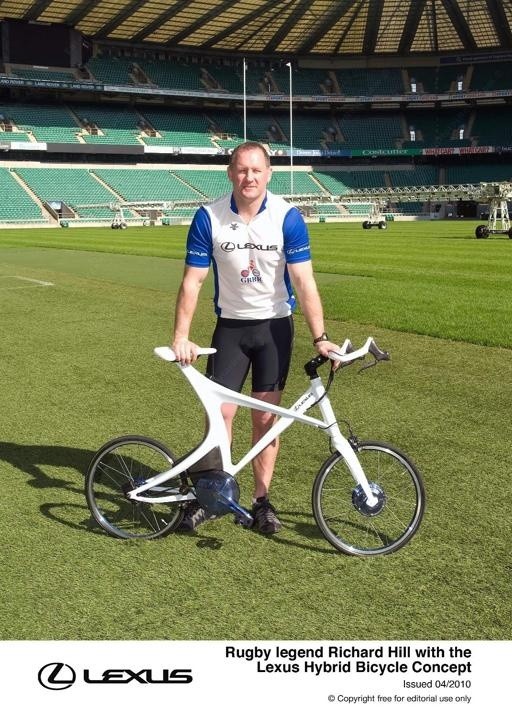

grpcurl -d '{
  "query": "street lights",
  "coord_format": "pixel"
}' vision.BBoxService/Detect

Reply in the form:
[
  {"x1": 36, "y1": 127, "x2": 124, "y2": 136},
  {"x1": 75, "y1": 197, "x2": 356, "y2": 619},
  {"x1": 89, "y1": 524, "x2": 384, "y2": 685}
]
[
  {"x1": 285, "y1": 60, "x2": 296, "y2": 197},
  {"x1": 241, "y1": 56, "x2": 248, "y2": 143}
]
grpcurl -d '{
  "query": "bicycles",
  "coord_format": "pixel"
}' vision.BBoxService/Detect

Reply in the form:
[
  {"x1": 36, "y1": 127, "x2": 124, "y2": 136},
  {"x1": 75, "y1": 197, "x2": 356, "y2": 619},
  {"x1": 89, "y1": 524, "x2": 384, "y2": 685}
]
[{"x1": 77, "y1": 331, "x2": 424, "y2": 558}]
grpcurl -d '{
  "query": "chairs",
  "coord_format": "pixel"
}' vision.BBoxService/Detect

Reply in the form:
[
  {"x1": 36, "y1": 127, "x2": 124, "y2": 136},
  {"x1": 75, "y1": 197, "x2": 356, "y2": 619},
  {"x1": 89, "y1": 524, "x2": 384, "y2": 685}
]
[{"x1": 1, "y1": 55, "x2": 511, "y2": 228}]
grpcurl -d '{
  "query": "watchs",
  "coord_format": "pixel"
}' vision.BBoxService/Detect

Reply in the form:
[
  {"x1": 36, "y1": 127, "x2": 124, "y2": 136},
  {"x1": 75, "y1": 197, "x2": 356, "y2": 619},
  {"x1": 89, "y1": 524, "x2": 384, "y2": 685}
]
[{"x1": 313, "y1": 332, "x2": 328, "y2": 346}]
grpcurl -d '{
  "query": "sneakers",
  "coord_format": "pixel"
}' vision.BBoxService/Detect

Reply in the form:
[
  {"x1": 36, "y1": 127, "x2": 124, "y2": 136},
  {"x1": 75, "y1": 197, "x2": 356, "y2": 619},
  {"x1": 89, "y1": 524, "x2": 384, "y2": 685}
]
[
  {"x1": 175, "y1": 498, "x2": 219, "y2": 534},
  {"x1": 248, "y1": 493, "x2": 285, "y2": 538}
]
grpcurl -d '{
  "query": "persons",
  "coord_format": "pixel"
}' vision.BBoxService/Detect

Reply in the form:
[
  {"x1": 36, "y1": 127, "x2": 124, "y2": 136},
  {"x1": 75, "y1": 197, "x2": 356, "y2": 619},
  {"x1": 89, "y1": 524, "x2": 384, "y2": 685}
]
[{"x1": 172, "y1": 143, "x2": 344, "y2": 531}]
